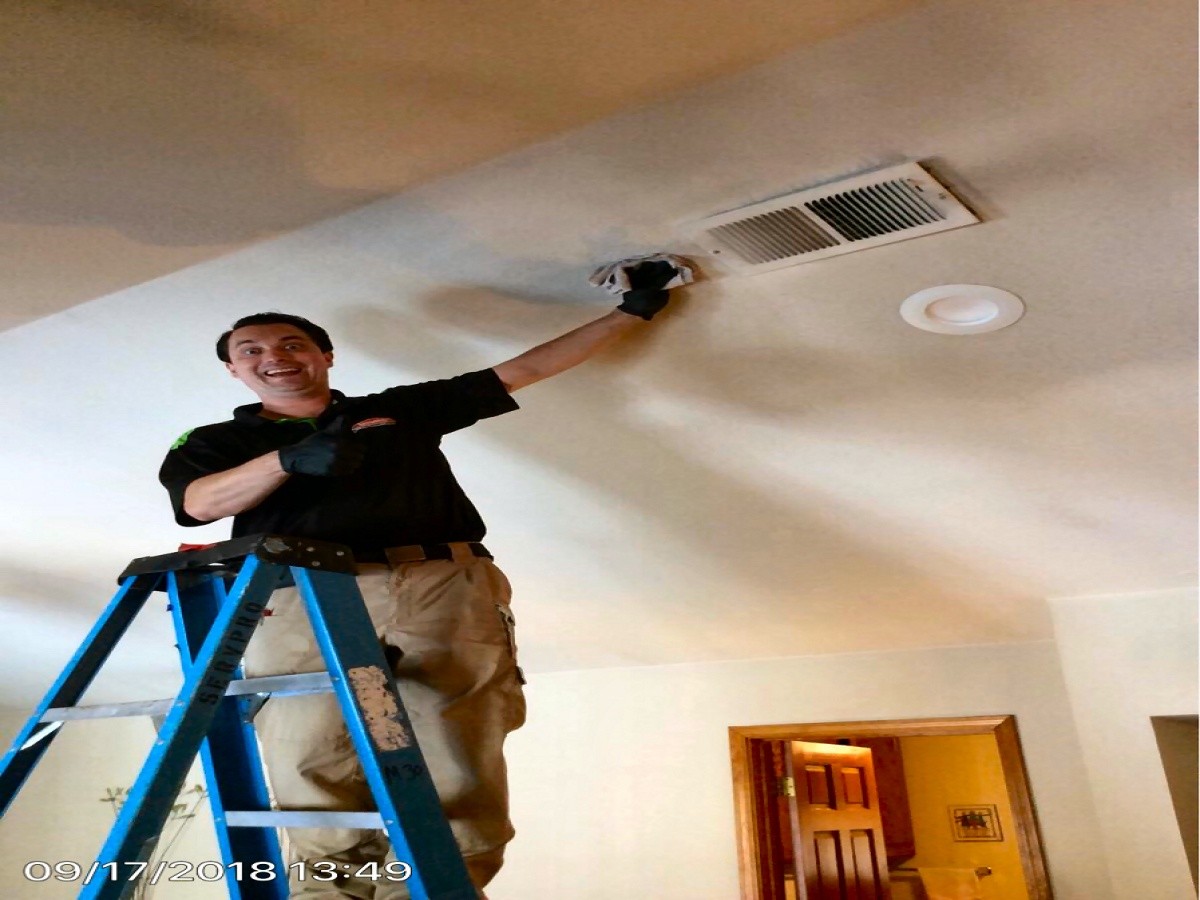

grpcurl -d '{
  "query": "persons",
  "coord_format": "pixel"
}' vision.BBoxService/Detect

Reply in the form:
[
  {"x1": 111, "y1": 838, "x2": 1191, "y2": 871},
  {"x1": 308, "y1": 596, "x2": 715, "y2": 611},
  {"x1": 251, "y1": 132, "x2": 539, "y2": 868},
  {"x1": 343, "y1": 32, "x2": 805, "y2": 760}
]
[{"x1": 159, "y1": 262, "x2": 681, "y2": 900}]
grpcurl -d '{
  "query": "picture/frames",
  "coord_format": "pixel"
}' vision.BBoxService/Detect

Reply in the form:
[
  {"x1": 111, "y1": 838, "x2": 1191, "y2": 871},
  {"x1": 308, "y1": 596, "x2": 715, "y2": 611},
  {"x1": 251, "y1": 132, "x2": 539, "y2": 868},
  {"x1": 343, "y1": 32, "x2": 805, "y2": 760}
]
[{"x1": 947, "y1": 804, "x2": 1003, "y2": 842}]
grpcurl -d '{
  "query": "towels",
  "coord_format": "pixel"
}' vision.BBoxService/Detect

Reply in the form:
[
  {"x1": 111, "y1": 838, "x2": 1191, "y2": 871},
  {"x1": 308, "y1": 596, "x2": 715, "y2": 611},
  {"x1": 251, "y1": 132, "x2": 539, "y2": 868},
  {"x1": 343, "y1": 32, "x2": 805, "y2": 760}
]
[{"x1": 917, "y1": 866, "x2": 985, "y2": 900}]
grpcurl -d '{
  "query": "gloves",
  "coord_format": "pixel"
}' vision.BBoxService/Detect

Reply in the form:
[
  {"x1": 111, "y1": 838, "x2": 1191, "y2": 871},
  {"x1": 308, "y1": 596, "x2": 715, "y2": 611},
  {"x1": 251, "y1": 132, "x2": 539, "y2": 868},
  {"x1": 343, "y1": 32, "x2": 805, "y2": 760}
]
[
  {"x1": 283, "y1": 429, "x2": 366, "y2": 477},
  {"x1": 610, "y1": 260, "x2": 683, "y2": 321}
]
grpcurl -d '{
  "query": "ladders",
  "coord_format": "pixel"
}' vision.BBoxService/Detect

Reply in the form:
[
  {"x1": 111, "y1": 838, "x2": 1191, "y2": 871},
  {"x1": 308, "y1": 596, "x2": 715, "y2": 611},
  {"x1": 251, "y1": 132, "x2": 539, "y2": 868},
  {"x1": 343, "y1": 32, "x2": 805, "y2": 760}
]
[{"x1": 1, "y1": 534, "x2": 482, "y2": 900}]
